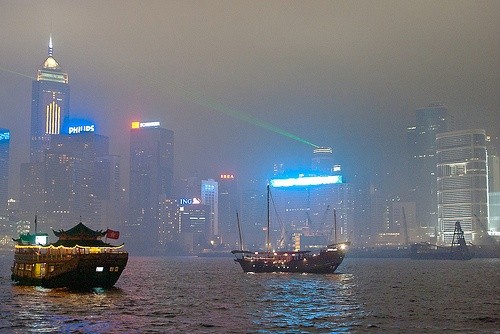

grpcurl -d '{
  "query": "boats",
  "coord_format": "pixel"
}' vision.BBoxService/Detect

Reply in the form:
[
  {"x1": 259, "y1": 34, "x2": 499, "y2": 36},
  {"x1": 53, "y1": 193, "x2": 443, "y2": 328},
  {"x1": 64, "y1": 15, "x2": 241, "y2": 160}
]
[
  {"x1": 409, "y1": 241, "x2": 472, "y2": 260},
  {"x1": 10, "y1": 214, "x2": 128, "y2": 289}
]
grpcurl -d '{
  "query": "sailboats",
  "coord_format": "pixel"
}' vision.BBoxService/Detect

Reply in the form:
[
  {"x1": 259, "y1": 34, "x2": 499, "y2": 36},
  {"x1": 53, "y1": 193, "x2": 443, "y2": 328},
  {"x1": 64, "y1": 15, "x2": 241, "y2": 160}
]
[
  {"x1": 230, "y1": 185, "x2": 351, "y2": 274},
  {"x1": 384, "y1": 206, "x2": 417, "y2": 258},
  {"x1": 469, "y1": 214, "x2": 500, "y2": 258}
]
